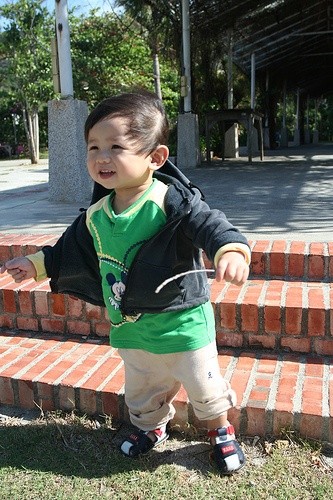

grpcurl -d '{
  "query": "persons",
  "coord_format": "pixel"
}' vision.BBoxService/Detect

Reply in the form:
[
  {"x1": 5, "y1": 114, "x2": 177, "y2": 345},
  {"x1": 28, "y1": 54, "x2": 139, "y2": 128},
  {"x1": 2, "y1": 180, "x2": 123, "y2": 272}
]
[{"x1": 0, "y1": 89, "x2": 252, "y2": 473}]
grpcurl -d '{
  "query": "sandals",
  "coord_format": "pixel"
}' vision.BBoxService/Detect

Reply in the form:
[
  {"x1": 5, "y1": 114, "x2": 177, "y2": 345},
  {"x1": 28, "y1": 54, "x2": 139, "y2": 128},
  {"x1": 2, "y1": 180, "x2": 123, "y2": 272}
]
[
  {"x1": 120, "y1": 420, "x2": 173, "y2": 457},
  {"x1": 208, "y1": 420, "x2": 245, "y2": 473}
]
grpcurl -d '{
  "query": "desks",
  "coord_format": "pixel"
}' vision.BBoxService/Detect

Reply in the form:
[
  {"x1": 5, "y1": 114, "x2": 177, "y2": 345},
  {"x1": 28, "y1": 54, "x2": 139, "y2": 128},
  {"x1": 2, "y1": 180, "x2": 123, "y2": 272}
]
[{"x1": 205, "y1": 108, "x2": 264, "y2": 163}]
